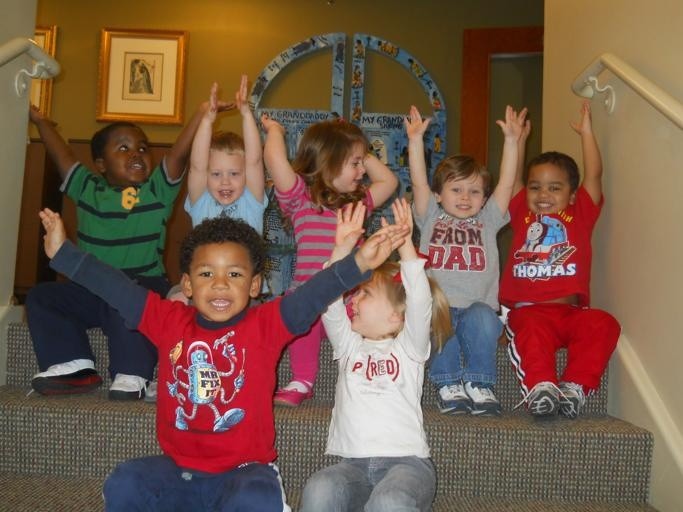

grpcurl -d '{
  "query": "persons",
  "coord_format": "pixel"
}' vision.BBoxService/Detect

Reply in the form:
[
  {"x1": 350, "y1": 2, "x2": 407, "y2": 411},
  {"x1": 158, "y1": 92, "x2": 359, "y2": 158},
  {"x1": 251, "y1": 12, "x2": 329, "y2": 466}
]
[
  {"x1": 260, "y1": 113, "x2": 400, "y2": 407},
  {"x1": 298, "y1": 196, "x2": 437, "y2": 512},
  {"x1": 24, "y1": 99, "x2": 211, "y2": 400},
  {"x1": 497, "y1": 99, "x2": 622, "y2": 417},
  {"x1": 144, "y1": 74, "x2": 270, "y2": 404},
  {"x1": 403, "y1": 103, "x2": 518, "y2": 416},
  {"x1": 38, "y1": 206, "x2": 410, "y2": 511}
]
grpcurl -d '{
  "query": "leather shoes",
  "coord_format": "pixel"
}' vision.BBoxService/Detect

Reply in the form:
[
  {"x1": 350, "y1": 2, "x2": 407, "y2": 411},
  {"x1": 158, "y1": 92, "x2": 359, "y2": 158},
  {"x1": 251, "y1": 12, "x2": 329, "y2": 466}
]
[{"x1": 272, "y1": 378, "x2": 314, "y2": 406}]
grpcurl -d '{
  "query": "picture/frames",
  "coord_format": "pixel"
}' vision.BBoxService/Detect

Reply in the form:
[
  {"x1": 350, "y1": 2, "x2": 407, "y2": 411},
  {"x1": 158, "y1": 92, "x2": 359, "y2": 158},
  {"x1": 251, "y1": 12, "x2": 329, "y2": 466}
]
[
  {"x1": 30, "y1": 24, "x2": 57, "y2": 118},
  {"x1": 95, "y1": 27, "x2": 191, "y2": 127}
]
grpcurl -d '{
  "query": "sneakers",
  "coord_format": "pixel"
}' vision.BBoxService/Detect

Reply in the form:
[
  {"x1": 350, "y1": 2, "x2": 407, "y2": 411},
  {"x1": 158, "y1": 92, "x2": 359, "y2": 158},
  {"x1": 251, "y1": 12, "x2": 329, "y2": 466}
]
[
  {"x1": 464, "y1": 381, "x2": 502, "y2": 416},
  {"x1": 436, "y1": 385, "x2": 473, "y2": 416},
  {"x1": 109, "y1": 373, "x2": 158, "y2": 403},
  {"x1": 32, "y1": 358, "x2": 103, "y2": 395},
  {"x1": 559, "y1": 384, "x2": 582, "y2": 419},
  {"x1": 528, "y1": 384, "x2": 559, "y2": 417}
]
